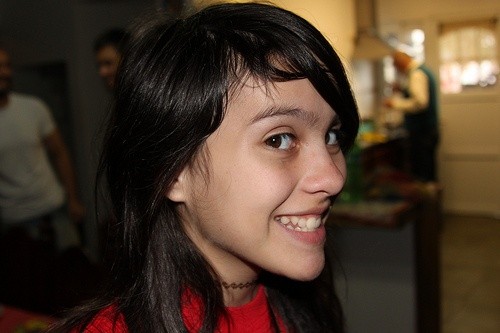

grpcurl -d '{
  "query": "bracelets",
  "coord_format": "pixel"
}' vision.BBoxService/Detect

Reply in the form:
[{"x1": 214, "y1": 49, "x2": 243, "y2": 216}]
[{"x1": 220, "y1": 281, "x2": 260, "y2": 289}]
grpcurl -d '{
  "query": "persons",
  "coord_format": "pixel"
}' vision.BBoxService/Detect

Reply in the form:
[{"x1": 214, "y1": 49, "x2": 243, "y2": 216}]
[
  {"x1": 44, "y1": 0, "x2": 361, "y2": 333},
  {"x1": 383, "y1": 51, "x2": 440, "y2": 187},
  {"x1": 0, "y1": 27, "x2": 134, "y2": 319}
]
